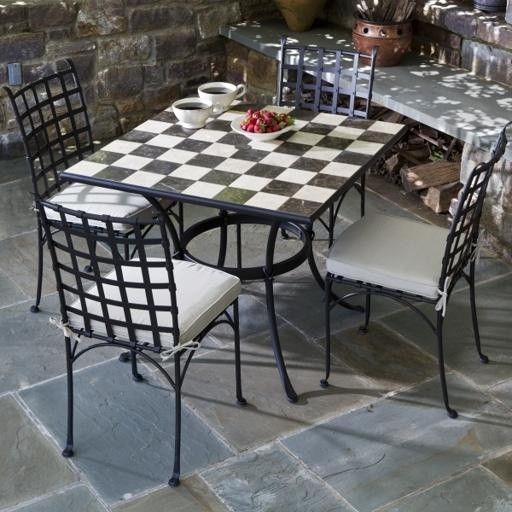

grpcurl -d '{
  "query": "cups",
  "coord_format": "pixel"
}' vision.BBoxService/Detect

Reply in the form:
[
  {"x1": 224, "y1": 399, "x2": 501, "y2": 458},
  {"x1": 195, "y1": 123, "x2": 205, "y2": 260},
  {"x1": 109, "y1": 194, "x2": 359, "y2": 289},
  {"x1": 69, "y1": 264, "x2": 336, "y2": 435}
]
[
  {"x1": 198, "y1": 81, "x2": 247, "y2": 111},
  {"x1": 172, "y1": 98, "x2": 223, "y2": 130}
]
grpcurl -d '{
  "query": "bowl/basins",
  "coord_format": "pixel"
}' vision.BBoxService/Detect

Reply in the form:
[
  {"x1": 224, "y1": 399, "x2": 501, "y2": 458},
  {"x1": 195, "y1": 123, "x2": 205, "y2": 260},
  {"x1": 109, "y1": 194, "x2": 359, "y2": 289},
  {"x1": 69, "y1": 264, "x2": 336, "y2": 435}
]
[{"x1": 230, "y1": 115, "x2": 293, "y2": 141}]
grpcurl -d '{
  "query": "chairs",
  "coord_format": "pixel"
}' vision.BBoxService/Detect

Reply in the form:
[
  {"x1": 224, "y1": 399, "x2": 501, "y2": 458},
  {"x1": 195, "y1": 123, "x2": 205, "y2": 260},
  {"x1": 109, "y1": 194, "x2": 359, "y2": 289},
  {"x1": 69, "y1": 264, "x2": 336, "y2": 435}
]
[
  {"x1": 318, "y1": 120, "x2": 512, "y2": 418},
  {"x1": 37, "y1": 191, "x2": 247, "y2": 486},
  {"x1": 274, "y1": 36, "x2": 379, "y2": 248},
  {"x1": 1, "y1": 59, "x2": 187, "y2": 315}
]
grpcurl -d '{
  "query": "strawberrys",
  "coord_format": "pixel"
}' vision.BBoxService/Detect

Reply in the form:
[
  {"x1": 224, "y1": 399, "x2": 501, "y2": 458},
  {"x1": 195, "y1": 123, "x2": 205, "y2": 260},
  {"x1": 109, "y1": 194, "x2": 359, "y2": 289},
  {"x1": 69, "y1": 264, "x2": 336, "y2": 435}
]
[{"x1": 241, "y1": 107, "x2": 295, "y2": 133}]
